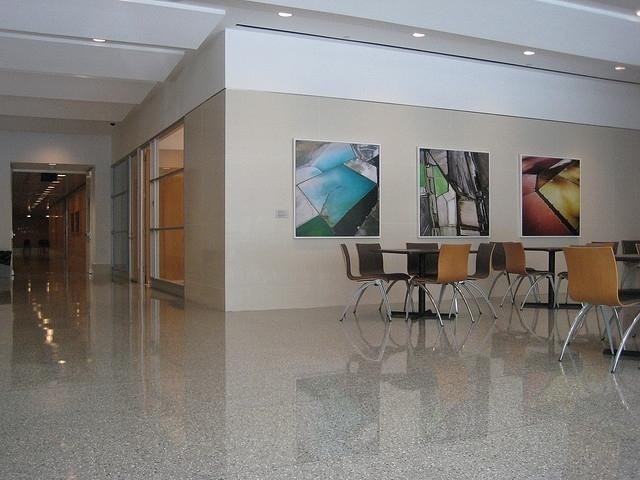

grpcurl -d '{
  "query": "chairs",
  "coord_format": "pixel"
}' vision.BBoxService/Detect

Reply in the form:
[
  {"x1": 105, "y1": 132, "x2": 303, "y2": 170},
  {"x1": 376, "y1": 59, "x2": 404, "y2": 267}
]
[{"x1": 0, "y1": 250, "x2": 16, "y2": 305}]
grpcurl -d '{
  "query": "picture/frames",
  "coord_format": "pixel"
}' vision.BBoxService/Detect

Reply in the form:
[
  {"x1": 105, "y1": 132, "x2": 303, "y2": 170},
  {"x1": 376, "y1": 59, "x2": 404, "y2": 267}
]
[
  {"x1": 416, "y1": 145, "x2": 492, "y2": 240},
  {"x1": 518, "y1": 154, "x2": 582, "y2": 239},
  {"x1": 292, "y1": 139, "x2": 382, "y2": 240}
]
